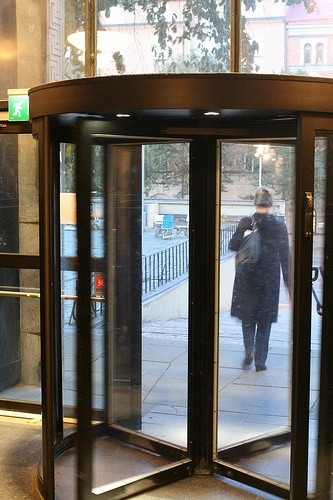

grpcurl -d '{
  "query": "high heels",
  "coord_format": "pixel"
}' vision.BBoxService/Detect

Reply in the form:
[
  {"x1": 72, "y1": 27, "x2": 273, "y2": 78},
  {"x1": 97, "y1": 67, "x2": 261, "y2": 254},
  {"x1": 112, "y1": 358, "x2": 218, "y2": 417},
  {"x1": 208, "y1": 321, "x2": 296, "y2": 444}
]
[
  {"x1": 255, "y1": 361, "x2": 267, "y2": 373},
  {"x1": 243, "y1": 351, "x2": 252, "y2": 370}
]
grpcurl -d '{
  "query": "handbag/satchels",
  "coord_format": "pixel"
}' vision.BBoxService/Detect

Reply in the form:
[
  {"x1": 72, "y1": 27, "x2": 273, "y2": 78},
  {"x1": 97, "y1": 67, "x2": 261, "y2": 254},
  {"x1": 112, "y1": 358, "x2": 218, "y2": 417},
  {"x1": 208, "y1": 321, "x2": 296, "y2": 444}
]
[{"x1": 236, "y1": 217, "x2": 260, "y2": 284}]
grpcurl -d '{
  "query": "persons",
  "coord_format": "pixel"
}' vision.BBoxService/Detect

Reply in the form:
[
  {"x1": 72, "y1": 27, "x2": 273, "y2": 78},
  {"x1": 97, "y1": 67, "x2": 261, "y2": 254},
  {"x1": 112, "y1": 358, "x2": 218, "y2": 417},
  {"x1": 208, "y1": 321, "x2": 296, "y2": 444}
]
[{"x1": 228, "y1": 186, "x2": 292, "y2": 371}]
